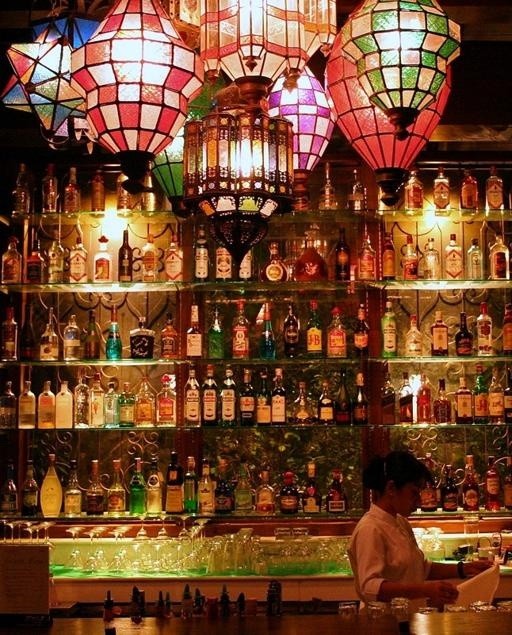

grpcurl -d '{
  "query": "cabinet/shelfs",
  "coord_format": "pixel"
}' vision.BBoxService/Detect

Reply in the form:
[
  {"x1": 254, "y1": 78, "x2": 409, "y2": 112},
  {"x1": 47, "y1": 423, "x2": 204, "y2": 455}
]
[
  {"x1": 367, "y1": 137, "x2": 511, "y2": 516},
  {"x1": 178, "y1": 127, "x2": 368, "y2": 521},
  {"x1": 1, "y1": 154, "x2": 175, "y2": 521}
]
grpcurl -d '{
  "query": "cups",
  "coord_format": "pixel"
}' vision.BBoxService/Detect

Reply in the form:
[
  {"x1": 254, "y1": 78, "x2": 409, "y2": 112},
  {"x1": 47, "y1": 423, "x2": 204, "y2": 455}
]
[
  {"x1": 477, "y1": 546, "x2": 490, "y2": 560},
  {"x1": 336, "y1": 596, "x2": 512, "y2": 619},
  {"x1": 194, "y1": 524, "x2": 353, "y2": 576}
]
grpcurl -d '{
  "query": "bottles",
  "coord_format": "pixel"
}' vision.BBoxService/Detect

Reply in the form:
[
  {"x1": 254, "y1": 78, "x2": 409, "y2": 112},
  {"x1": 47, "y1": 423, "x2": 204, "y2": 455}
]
[
  {"x1": 93, "y1": 236, "x2": 113, "y2": 284},
  {"x1": 484, "y1": 165, "x2": 505, "y2": 209},
  {"x1": 234, "y1": 457, "x2": 254, "y2": 514},
  {"x1": 129, "y1": 316, "x2": 156, "y2": 360},
  {"x1": 115, "y1": 171, "x2": 131, "y2": 209},
  {"x1": 68, "y1": 236, "x2": 89, "y2": 284},
  {"x1": 378, "y1": 301, "x2": 397, "y2": 359},
  {"x1": 200, "y1": 364, "x2": 218, "y2": 426},
  {"x1": 89, "y1": 372, "x2": 105, "y2": 428},
  {"x1": 215, "y1": 458, "x2": 234, "y2": 515},
  {"x1": 285, "y1": 381, "x2": 318, "y2": 427},
  {"x1": 154, "y1": 375, "x2": 177, "y2": 429},
  {"x1": 37, "y1": 307, "x2": 59, "y2": 362},
  {"x1": 380, "y1": 372, "x2": 395, "y2": 425},
  {"x1": 396, "y1": 371, "x2": 413, "y2": 424},
  {"x1": 504, "y1": 372, "x2": 512, "y2": 425},
  {"x1": 0, "y1": 378, "x2": 16, "y2": 430},
  {"x1": 281, "y1": 300, "x2": 300, "y2": 360},
  {"x1": 215, "y1": 241, "x2": 233, "y2": 281},
  {"x1": 21, "y1": 459, "x2": 39, "y2": 518},
  {"x1": 90, "y1": 168, "x2": 106, "y2": 211},
  {"x1": 39, "y1": 162, "x2": 58, "y2": 214},
  {"x1": 460, "y1": 454, "x2": 480, "y2": 511},
  {"x1": 453, "y1": 376, "x2": 474, "y2": 425},
  {"x1": 502, "y1": 455, "x2": 512, "y2": 511},
  {"x1": 72, "y1": 365, "x2": 91, "y2": 430},
  {"x1": 501, "y1": 303, "x2": 512, "y2": 357},
  {"x1": 326, "y1": 468, "x2": 346, "y2": 514},
  {"x1": 255, "y1": 369, "x2": 271, "y2": 426},
  {"x1": 402, "y1": 234, "x2": 419, "y2": 281},
  {"x1": 476, "y1": 301, "x2": 493, "y2": 356},
  {"x1": 16, "y1": 380, "x2": 37, "y2": 430},
  {"x1": 302, "y1": 461, "x2": 322, "y2": 515},
  {"x1": 1, "y1": 306, "x2": 18, "y2": 362},
  {"x1": 377, "y1": 186, "x2": 396, "y2": 211},
  {"x1": 0, "y1": 458, "x2": 19, "y2": 516},
  {"x1": 46, "y1": 229, "x2": 64, "y2": 284},
  {"x1": 13, "y1": 162, "x2": 35, "y2": 216},
  {"x1": 316, "y1": 376, "x2": 336, "y2": 427},
  {"x1": 63, "y1": 459, "x2": 82, "y2": 519},
  {"x1": 142, "y1": 233, "x2": 158, "y2": 283},
  {"x1": 346, "y1": 165, "x2": 368, "y2": 211},
  {"x1": 482, "y1": 455, "x2": 499, "y2": 512},
  {"x1": 488, "y1": 235, "x2": 510, "y2": 281},
  {"x1": 165, "y1": 230, "x2": 183, "y2": 283},
  {"x1": 63, "y1": 314, "x2": 81, "y2": 363},
  {"x1": 182, "y1": 368, "x2": 202, "y2": 429},
  {"x1": 162, "y1": 194, "x2": 173, "y2": 211},
  {"x1": 128, "y1": 456, "x2": 147, "y2": 519},
  {"x1": 145, "y1": 454, "x2": 163, "y2": 520},
  {"x1": 103, "y1": 577, "x2": 284, "y2": 620},
  {"x1": 333, "y1": 226, "x2": 351, "y2": 281},
  {"x1": 354, "y1": 233, "x2": 376, "y2": 281},
  {"x1": 133, "y1": 377, "x2": 155, "y2": 430},
  {"x1": 350, "y1": 368, "x2": 368, "y2": 426},
  {"x1": 1, "y1": 230, "x2": 23, "y2": 283},
  {"x1": 454, "y1": 310, "x2": 473, "y2": 356},
  {"x1": 230, "y1": 300, "x2": 251, "y2": 361},
  {"x1": 118, "y1": 381, "x2": 135, "y2": 429},
  {"x1": 318, "y1": 160, "x2": 337, "y2": 211},
  {"x1": 106, "y1": 459, "x2": 126, "y2": 519},
  {"x1": 219, "y1": 364, "x2": 237, "y2": 427},
  {"x1": 324, "y1": 306, "x2": 347, "y2": 359},
  {"x1": 185, "y1": 302, "x2": 204, "y2": 360},
  {"x1": 207, "y1": 302, "x2": 226, "y2": 360},
  {"x1": 353, "y1": 301, "x2": 370, "y2": 360},
  {"x1": 466, "y1": 238, "x2": 483, "y2": 281},
  {"x1": 39, "y1": 453, "x2": 63, "y2": 519},
  {"x1": 166, "y1": 451, "x2": 184, "y2": 515},
  {"x1": 159, "y1": 312, "x2": 178, "y2": 360},
  {"x1": 198, "y1": 461, "x2": 215, "y2": 514},
  {"x1": 304, "y1": 299, "x2": 324, "y2": 360},
  {"x1": 430, "y1": 309, "x2": 448, "y2": 356},
  {"x1": 61, "y1": 166, "x2": 82, "y2": 214},
  {"x1": 83, "y1": 458, "x2": 105, "y2": 519},
  {"x1": 259, "y1": 240, "x2": 288, "y2": 283},
  {"x1": 255, "y1": 461, "x2": 275, "y2": 515},
  {"x1": 55, "y1": 381, "x2": 73, "y2": 430},
  {"x1": 105, "y1": 303, "x2": 122, "y2": 360},
  {"x1": 18, "y1": 303, "x2": 35, "y2": 361},
  {"x1": 402, "y1": 166, "x2": 423, "y2": 213},
  {"x1": 334, "y1": 366, "x2": 352, "y2": 426},
  {"x1": 486, "y1": 365, "x2": 504, "y2": 425},
  {"x1": 459, "y1": 164, "x2": 479, "y2": 209},
  {"x1": 184, "y1": 456, "x2": 198, "y2": 513},
  {"x1": 431, "y1": 377, "x2": 452, "y2": 425},
  {"x1": 116, "y1": 229, "x2": 134, "y2": 283},
  {"x1": 35, "y1": 380, "x2": 56, "y2": 430},
  {"x1": 258, "y1": 301, "x2": 278, "y2": 361},
  {"x1": 402, "y1": 315, "x2": 422, "y2": 359},
  {"x1": 440, "y1": 464, "x2": 458, "y2": 512},
  {"x1": 415, "y1": 372, "x2": 432, "y2": 424},
  {"x1": 420, "y1": 452, "x2": 437, "y2": 512},
  {"x1": 473, "y1": 363, "x2": 489, "y2": 424},
  {"x1": 292, "y1": 231, "x2": 328, "y2": 282},
  {"x1": 443, "y1": 234, "x2": 463, "y2": 281},
  {"x1": 238, "y1": 367, "x2": 256, "y2": 427},
  {"x1": 270, "y1": 366, "x2": 286, "y2": 427},
  {"x1": 102, "y1": 381, "x2": 119, "y2": 429},
  {"x1": 423, "y1": 237, "x2": 440, "y2": 280},
  {"x1": 141, "y1": 175, "x2": 155, "y2": 211},
  {"x1": 237, "y1": 246, "x2": 254, "y2": 281},
  {"x1": 192, "y1": 225, "x2": 210, "y2": 282},
  {"x1": 431, "y1": 164, "x2": 451, "y2": 210},
  {"x1": 85, "y1": 309, "x2": 101, "y2": 360},
  {"x1": 278, "y1": 470, "x2": 299, "y2": 514},
  {"x1": 380, "y1": 231, "x2": 396, "y2": 280},
  {"x1": 24, "y1": 239, "x2": 44, "y2": 284}
]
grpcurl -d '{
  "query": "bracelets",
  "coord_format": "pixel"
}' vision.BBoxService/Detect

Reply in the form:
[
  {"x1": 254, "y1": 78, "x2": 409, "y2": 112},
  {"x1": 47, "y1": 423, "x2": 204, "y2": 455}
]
[{"x1": 457, "y1": 562, "x2": 464, "y2": 579}]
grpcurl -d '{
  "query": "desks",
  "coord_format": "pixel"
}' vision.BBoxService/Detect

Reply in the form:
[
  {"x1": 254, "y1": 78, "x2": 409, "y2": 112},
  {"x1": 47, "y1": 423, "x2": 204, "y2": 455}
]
[{"x1": 48, "y1": 613, "x2": 512, "y2": 635}]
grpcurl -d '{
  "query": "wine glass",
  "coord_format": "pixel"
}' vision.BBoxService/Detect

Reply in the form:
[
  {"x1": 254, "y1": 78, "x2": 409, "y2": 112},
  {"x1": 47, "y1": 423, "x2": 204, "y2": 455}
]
[
  {"x1": 63, "y1": 515, "x2": 209, "y2": 577},
  {"x1": 410, "y1": 527, "x2": 445, "y2": 563},
  {"x1": 0, "y1": 520, "x2": 56, "y2": 571}
]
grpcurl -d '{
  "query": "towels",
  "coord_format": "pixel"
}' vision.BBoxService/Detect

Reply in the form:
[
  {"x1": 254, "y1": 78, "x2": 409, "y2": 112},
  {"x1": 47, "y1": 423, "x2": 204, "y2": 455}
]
[{"x1": 444, "y1": 564, "x2": 500, "y2": 612}]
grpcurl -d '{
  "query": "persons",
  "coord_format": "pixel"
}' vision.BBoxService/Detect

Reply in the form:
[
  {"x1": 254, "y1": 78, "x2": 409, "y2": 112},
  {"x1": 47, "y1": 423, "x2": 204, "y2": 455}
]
[{"x1": 346, "y1": 451, "x2": 498, "y2": 604}]
